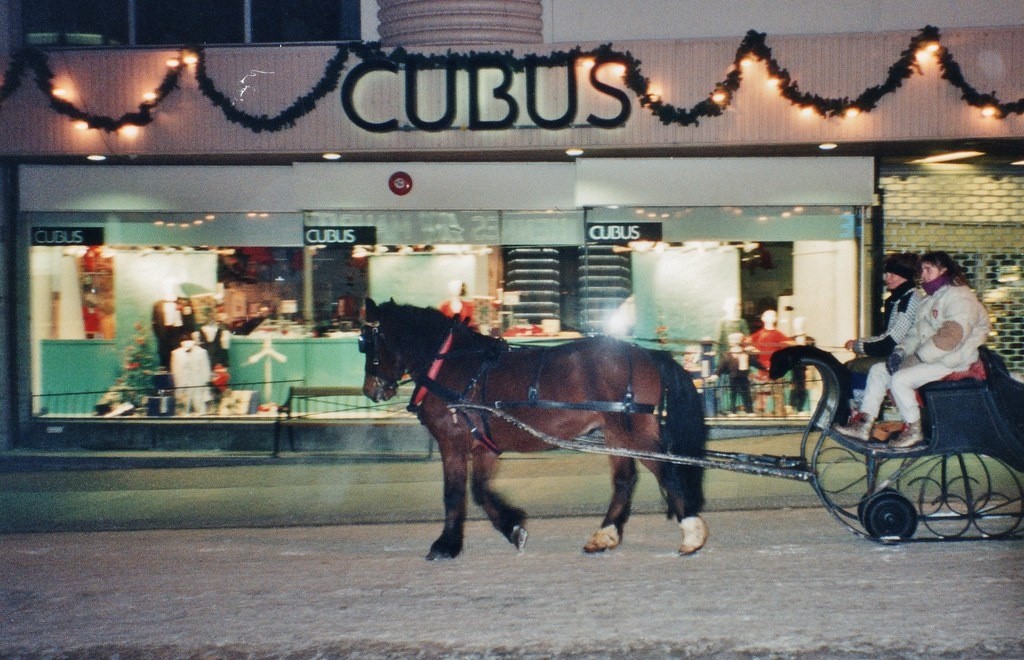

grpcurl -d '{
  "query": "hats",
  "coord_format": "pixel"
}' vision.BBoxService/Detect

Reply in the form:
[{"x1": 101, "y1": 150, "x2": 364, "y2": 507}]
[{"x1": 886, "y1": 253, "x2": 918, "y2": 281}]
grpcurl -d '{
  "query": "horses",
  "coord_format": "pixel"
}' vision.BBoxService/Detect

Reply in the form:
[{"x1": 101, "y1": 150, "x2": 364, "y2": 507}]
[{"x1": 354, "y1": 295, "x2": 710, "y2": 564}]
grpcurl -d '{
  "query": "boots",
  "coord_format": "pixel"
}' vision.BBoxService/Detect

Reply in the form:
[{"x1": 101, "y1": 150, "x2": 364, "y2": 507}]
[
  {"x1": 834, "y1": 416, "x2": 874, "y2": 442},
  {"x1": 889, "y1": 417, "x2": 924, "y2": 448}
]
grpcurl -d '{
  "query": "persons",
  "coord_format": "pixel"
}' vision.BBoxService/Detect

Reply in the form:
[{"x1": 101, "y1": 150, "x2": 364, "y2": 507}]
[
  {"x1": 153, "y1": 279, "x2": 230, "y2": 416},
  {"x1": 835, "y1": 250, "x2": 990, "y2": 448},
  {"x1": 718, "y1": 310, "x2": 815, "y2": 416},
  {"x1": 843, "y1": 252, "x2": 923, "y2": 419},
  {"x1": 440, "y1": 280, "x2": 475, "y2": 328}
]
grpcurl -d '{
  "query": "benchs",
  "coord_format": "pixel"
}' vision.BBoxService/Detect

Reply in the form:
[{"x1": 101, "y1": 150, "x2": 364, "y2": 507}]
[
  {"x1": 269, "y1": 387, "x2": 434, "y2": 457},
  {"x1": 917, "y1": 378, "x2": 989, "y2": 445}
]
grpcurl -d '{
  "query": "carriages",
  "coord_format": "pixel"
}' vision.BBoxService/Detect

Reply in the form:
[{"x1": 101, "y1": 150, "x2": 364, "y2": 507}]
[{"x1": 357, "y1": 294, "x2": 1022, "y2": 562}]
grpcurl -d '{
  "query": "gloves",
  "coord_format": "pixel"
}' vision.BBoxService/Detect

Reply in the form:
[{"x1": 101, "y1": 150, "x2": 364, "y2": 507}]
[
  {"x1": 901, "y1": 354, "x2": 921, "y2": 368},
  {"x1": 886, "y1": 349, "x2": 906, "y2": 376}
]
